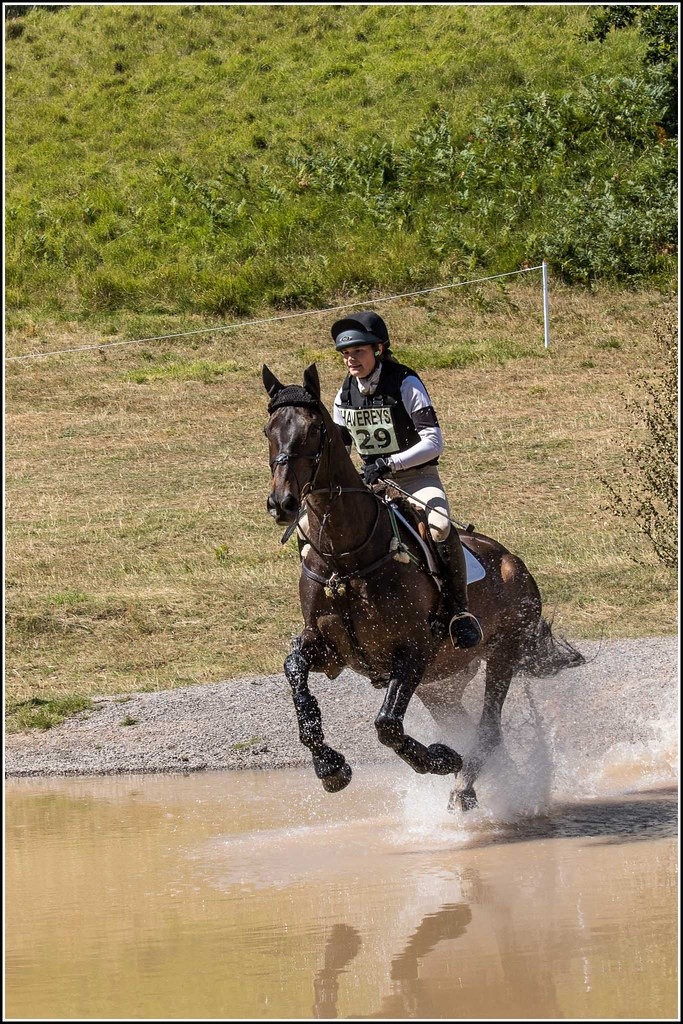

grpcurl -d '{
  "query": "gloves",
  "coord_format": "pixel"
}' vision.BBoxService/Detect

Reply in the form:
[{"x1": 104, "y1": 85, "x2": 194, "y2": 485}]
[{"x1": 364, "y1": 457, "x2": 392, "y2": 485}]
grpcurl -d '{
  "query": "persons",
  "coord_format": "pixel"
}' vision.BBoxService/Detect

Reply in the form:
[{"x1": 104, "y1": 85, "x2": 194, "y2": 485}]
[{"x1": 331, "y1": 312, "x2": 480, "y2": 648}]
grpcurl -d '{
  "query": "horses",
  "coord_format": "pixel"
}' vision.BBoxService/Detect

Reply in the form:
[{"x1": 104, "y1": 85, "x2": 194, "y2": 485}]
[{"x1": 258, "y1": 360, "x2": 605, "y2": 815}]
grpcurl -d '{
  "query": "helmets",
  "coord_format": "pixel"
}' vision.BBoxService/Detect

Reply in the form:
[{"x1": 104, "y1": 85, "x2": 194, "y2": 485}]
[{"x1": 330, "y1": 311, "x2": 394, "y2": 354}]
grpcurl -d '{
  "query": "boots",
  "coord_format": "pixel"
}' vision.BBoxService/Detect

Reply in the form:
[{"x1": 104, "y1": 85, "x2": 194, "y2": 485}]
[{"x1": 426, "y1": 522, "x2": 482, "y2": 649}]
